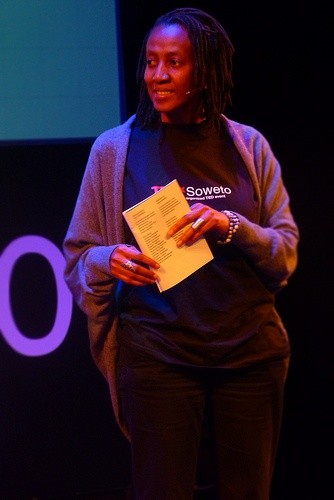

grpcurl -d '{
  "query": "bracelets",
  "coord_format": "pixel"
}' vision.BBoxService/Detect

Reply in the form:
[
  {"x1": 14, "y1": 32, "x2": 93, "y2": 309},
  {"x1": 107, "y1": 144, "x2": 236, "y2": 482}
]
[{"x1": 218, "y1": 210, "x2": 240, "y2": 245}]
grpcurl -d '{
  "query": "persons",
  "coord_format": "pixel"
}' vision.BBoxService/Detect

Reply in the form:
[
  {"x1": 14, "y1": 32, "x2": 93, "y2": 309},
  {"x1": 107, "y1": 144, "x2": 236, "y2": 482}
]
[{"x1": 62, "y1": 9, "x2": 300, "y2": 500}]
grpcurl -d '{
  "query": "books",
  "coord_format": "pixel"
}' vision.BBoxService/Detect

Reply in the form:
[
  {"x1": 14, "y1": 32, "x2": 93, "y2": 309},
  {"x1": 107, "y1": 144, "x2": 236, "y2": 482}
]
[{"x1": 121, "y1": 177, "x2": 216, "y2": 294}]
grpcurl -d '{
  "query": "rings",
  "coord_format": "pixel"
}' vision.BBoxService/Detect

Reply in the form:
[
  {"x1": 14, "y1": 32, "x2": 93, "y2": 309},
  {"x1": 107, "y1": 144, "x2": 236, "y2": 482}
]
[
  {"x1": 123, "y1": 260, "x2": 133, "y2": 270},
  {"x1": 192, "y1": 218, "x2": 204, "y2": 230}
]
